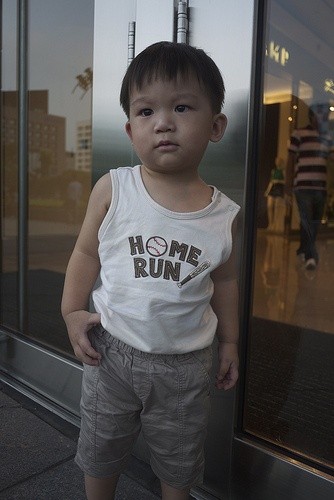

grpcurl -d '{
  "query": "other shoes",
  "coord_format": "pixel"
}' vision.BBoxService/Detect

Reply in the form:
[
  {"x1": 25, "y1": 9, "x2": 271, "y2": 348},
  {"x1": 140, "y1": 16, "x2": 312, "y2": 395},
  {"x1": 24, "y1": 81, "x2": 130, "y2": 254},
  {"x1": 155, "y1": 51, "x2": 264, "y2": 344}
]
[{"x1": 301, "y1": 253, "x2": 316, "y2": 269}]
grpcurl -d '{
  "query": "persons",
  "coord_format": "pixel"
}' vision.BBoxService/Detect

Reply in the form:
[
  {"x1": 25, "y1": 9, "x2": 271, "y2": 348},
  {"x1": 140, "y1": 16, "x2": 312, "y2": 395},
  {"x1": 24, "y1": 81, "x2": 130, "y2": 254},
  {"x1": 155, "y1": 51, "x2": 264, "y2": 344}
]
[
  {"x1": 264, "y1": 157, "x2": 287, "y2": 232},
  {"x1": 286, "y1": 99, "x2": 334, "y2": 268},
  {"x1": 61, "y1": 41, "x2": 241, "y2": 500}
]
